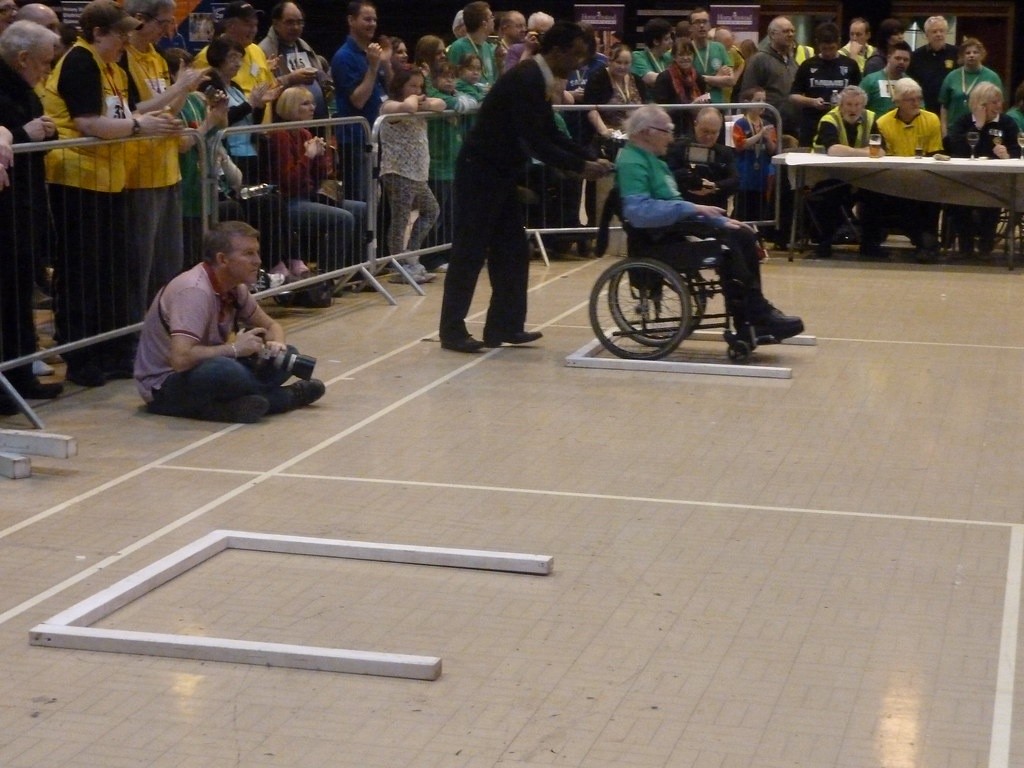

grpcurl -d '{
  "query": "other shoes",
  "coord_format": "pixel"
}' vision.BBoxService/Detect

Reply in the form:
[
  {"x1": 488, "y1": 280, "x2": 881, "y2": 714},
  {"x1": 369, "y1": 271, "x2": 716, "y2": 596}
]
[
  {"x1": 280, "y1": 378, "x2": 325, "y2": 407},
  {"x1": 28, "y1": 362, "x2": 55, "y2": 375},
  {"x1": 222, "y1": 396, "x2": 269, "y2": 422},
  {"x1": 734, "y1": 304, "x2": 803, "y2": 340},
  {"x1": 22, "y1": 381, "x2": 62, "y2": 399},
  {"x1": 32, "y1": 291, "x2": 53, "y2": 309},
  {"x1": 280, "y1": 258, "x2": 452, "y2": 307},
  {"x1": 529, "y1": 228, "x2": 598, "y2": 262},
  {"x1": 65, "y1": 364, "x2": 104, "y2": 387},
  {"x1": 0, "y1": 391, "x2": 18, "y2": 416},
  {"x1": 810, "y1": 233, "x2": 991, "y2": 263},
  {"x1": 42, "y1": 354, "x2": 64, "y2": 363}
]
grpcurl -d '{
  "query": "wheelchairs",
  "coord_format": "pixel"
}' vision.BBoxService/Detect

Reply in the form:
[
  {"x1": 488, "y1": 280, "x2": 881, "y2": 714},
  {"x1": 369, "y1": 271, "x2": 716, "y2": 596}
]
[{"x1": 589, "y1": 186, "x2": 781, "y2": 365}]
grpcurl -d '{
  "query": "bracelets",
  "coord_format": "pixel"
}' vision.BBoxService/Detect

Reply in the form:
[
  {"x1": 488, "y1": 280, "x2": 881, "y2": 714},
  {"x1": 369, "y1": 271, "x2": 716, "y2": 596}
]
[{"x1": 230, "y1": 342, "x2": 238, "y2": 358}]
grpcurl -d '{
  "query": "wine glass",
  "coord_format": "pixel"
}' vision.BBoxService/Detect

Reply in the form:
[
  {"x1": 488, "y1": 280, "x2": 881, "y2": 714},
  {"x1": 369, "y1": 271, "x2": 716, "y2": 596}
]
[
  {"x1": 967, "y1": 132, "x2": 979, "y2": 158},
  {"x1": 1017, "y1": 131, "x2": 1024, "y2": 161}
]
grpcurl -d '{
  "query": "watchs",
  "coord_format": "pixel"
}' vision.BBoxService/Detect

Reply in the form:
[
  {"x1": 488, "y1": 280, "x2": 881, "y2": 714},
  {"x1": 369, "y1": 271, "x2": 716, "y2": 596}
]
[{"x1": 132, "y1": 118, "x2": 141, "y2": 135}]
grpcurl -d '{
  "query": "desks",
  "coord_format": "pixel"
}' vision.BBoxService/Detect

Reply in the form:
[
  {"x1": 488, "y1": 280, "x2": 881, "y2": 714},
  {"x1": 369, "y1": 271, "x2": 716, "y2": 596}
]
[{"x1": 771, "y1": 152, "x2": 1024, "y2": 271}]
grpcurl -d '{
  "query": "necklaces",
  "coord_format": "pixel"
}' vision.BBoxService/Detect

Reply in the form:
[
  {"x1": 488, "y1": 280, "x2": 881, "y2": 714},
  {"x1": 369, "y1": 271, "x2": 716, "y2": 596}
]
[
  {"x1": 647, "y1": 48, "x2": 665, "y2": 73},
  {"x1": 465, "y1": 33, "x2": 486, "y2": 77},
  {"x1": 127, "y1": 44, "x2": 162, "y2": 93},
  {"x1": 961, "y1": 64, "x2": 980, "y2": 98},
  {"x1": 691, "y1": 39, "x2": 710, "y2": 73},
  {"x1": 613, "y1": 77, "x2": 630, "y2": 104},
  {"x1": 499, "y1": 36, "x2": 509, "y2": 50},
  {"x1": 576, "y1": 65, "x2": 588, "y2": 84},
  {"x1": 224, "y1": 82, "x2": 249, "y2": 122},
  {"x1": 887, "y1": 70, "x2": 906, "y2": 103}
]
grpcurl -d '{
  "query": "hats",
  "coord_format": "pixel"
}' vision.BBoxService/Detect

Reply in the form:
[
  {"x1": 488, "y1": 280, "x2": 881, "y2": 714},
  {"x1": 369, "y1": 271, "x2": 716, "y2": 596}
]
[
  {"x1": 223, "y1": 2, "x2": 263, "y2": 19},
  {"x1": 79, "y1": 0, "x2": 143, "y2": 33}
]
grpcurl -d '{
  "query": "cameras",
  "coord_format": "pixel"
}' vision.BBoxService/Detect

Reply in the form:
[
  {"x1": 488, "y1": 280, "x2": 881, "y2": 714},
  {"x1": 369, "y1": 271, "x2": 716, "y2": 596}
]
[
  {"x1": 205, "y1": 86, "x2": 220, "y2": 102},
  {"x1": 244, "y1": 332, "x2": 317, "y2": 382}
]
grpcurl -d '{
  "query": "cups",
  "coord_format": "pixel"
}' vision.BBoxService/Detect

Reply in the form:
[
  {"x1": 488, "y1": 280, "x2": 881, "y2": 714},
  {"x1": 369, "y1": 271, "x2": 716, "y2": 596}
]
[
  {"x1": 868, "y1": 134, "x2": 882, "y2": 156},
  {"x1": 915, "y1": 135, "x2": 923, "y2": 157}
]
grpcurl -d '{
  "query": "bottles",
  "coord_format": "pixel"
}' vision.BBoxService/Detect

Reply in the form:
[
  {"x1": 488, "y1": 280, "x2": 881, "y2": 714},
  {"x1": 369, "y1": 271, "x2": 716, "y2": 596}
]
[{"x1": 240, "y1": 182, "x2": 277, "y2": 200}]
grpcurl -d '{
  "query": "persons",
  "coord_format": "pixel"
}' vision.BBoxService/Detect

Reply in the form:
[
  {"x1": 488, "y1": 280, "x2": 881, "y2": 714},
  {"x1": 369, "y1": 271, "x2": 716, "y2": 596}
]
[
  {"x1": 134, "y1": 220, "x2": 326, "y2": 424},
  {"x1": 438, "y1": 22, "x2": 612, "y2": 353},
  {"x1": 0, "y1": 0, "x2": 1024, "y2": 417},
  {"x1": 616, "y1": 107, "x2": 804, "y2": 345}
]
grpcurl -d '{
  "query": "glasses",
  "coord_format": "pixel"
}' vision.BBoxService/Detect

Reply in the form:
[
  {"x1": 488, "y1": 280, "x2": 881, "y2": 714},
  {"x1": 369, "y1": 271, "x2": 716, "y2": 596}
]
[
  {"x1": 144, "y1": 12, "x2": 175, "y2": 28},
  {"x1": 689, "y1": 19, "x2": 709, "y2": 26},
  {"x1": 277, "y1": 20, "x2": 305, "y2": 27},
  {"x1": 483, "y1": 16, "x2": 496, "y2": 23},
  {"x1": 649, "y1": 126, "x2": 675, "y2": 136}
]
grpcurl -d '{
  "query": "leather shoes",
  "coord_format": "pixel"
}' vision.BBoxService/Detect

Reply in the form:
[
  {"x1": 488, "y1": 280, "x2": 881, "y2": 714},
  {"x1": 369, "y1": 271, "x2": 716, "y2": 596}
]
[
  {"x1": 440, "y1": 334, "x2": 483, "y2": 352},
  {"x1": 484, "y1": 330, "x2": 542, "y2": 347}
]
